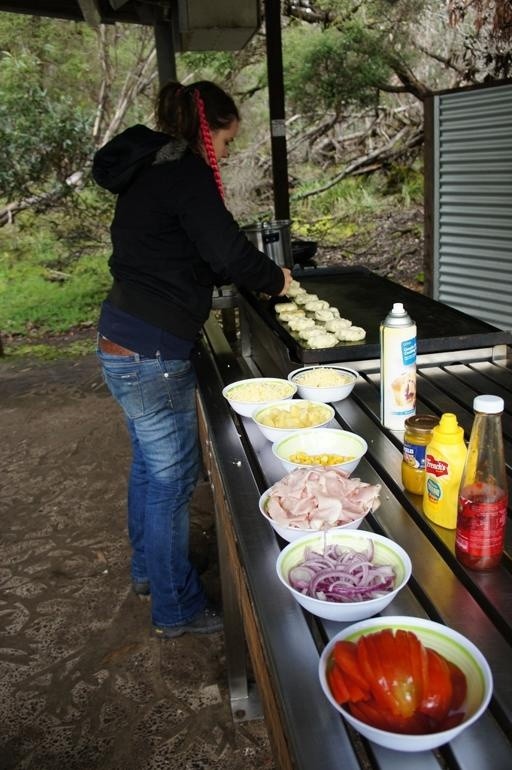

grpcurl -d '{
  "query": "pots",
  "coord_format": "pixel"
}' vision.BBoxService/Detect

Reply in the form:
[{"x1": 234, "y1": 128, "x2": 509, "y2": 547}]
[{"x1": 241, "y1": 218, "x2": 296, "y2": 270}]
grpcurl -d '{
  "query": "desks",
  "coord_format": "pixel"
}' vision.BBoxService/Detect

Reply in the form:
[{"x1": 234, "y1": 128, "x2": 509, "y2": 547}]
[{"x1": 194, "y1": 302, "x2": 512, "y2": 770}]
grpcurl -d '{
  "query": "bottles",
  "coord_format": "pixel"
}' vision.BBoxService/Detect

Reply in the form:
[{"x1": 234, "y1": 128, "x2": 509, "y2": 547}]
[
  {"x1": 455, "y1": 394, "x2": 509, "y2": 573},
  {"x1": 378, "y1": 303, "x2": 419, "y2": 431}
]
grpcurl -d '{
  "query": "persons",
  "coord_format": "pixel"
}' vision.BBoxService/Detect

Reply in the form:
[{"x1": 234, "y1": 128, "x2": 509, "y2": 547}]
[{"x1": 95, "y1": 78, "x2": 293, "y2": 640}]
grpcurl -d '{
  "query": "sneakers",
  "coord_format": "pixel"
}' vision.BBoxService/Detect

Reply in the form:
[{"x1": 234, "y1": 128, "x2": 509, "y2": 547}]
[
  {"x1": 133, "y1": 581, "x2": 152, "y2": 596},
  {"x1": 151, "y1": 604, "x2": 223, "y2": 639}
]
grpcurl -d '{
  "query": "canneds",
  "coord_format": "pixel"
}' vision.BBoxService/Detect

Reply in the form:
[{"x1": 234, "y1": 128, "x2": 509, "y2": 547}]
[{"x1": 401, "y1": 414, "x2": 441, "y2": 495}]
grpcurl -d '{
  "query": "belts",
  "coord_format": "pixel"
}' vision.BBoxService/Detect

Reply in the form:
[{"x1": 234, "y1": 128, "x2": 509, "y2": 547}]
[{"x1": 98, "y1": 335, "x2": 137, "y2": 357}]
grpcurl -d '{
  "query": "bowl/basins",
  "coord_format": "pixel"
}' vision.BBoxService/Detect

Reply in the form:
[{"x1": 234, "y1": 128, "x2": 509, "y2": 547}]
[
  {"x1": 286, "y1": 366, "x2": 360, "y2": 403},
  {"x1": 319, "y1": 615, "x2": 494, "y2": 752},
  {"x1": 270, "y1": 427, "x2": 369, "y2": 479},
  {"x1": 274, "y1": 528, "x2": 413, "y2": 621},
  {"x1": 257, "y1": 477, "x2": 372, "y2": 542},
  {"x1": 250, "y1": 400, "x2": 336, "y2": 442},
  {"x1": 224, "y1": 377, "x2": 298, "y2": 419},
  {"x1": 288, "y1": 240, "x2": 317, "y2": 261}
]
